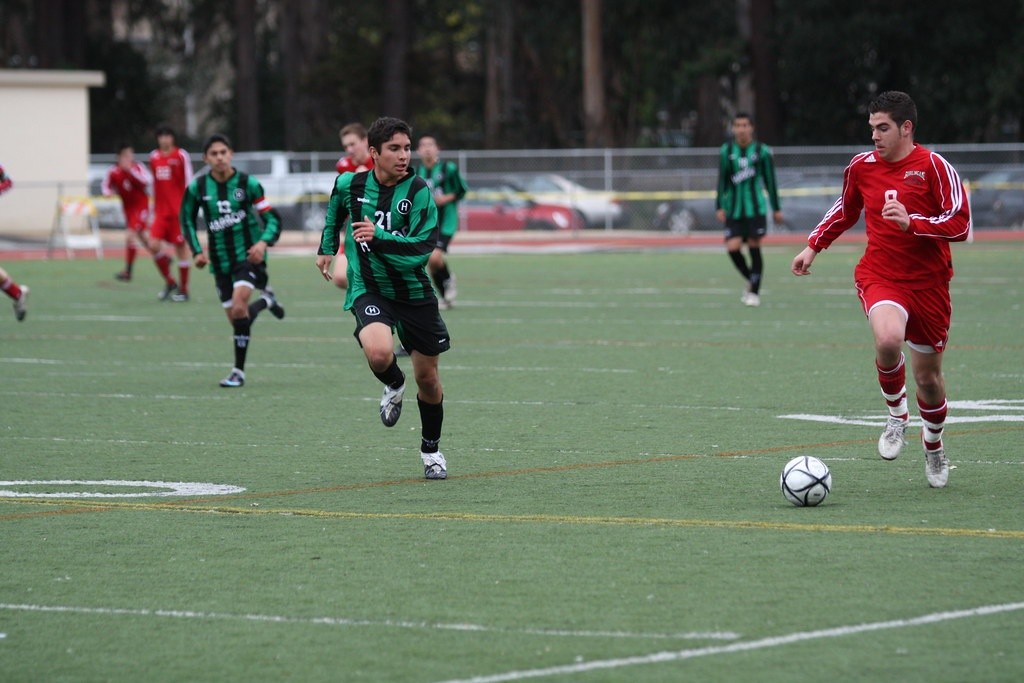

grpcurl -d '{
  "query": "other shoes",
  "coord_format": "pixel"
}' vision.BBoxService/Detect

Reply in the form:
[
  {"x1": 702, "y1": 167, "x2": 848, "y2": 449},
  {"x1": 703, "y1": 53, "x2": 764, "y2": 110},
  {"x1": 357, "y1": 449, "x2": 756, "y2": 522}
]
[
  {"x1": 171, "y1": 292, "x2": 188, "y2": 301},
  {"x1": 114, "y1": 271, "x2": 131, "y2": 280},
  {"x1": 159, "y1": 284, "x2": 177, "y2": 300},
  {"x1": 741, "y1": 283, "x2": 760, "y2": 307},
  {"x1": 442, "y1": 273, "x2": 458, "y2": 304}
]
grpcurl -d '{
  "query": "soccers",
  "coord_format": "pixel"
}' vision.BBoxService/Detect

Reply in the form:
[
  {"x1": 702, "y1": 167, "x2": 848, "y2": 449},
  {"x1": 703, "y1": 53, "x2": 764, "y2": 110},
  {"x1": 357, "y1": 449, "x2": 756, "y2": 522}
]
[{"x1": 781, "y1": 455, "x2": 831, "y2": 507}]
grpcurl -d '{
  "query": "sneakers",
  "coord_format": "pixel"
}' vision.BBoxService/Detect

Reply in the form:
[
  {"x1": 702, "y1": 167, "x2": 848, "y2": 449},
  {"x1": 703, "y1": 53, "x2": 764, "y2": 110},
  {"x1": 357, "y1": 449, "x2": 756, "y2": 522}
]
[
  {"x1": 14, "y1": 285, "x2": 29, "y2": 322},
  {"x1": 219, "y1": 366, "x2": 245, "y2": 387},
  {"x1": 420, "y1": 452, "x2": 447, "y2": 480},
  {"x1": 921, "y1": 430, "x2": 949, "y2": 488},
  {"x1": 380, "y1": 373, "x2": 407, "y2": 428},
  {"x1": 261, "y1": 288, "x2": 285, "y2": 319},
  {"x1": 878, "y1": 415, "x2": 910, "y2": 460}
]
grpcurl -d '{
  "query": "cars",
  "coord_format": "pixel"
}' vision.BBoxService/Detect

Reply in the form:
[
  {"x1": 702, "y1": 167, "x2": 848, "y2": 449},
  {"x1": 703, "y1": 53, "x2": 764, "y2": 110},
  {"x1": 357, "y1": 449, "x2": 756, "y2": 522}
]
[
  {"x1": 458, "y1": 171, "x2": 625, "y2": 232},
  {"x1": 657, "y1": 168, "x2": 856, "y2": 233},
  {"x1": 87, "y1": 161, "x2": 157, "y2": 238},
  {"x1": 969, "y1": 165, "x2": 1024, "y2": 228}
]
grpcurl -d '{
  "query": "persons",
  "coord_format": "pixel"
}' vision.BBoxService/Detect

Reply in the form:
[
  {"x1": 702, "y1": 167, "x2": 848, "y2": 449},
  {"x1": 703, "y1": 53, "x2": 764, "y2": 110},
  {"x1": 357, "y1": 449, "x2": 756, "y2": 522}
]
[
  {"x1": 178, "y1": 132, "x2": 285, "y2": 388},
  {"x1": 0, "y1": 164, "x2": 30, "y2": 322},
  {"x1": 316, "y1": 119, "x2": 451, "y2": 479},
  {"x1": 411, "y1": 132, "x2": 468, "y2": 309},
  {"x1": 713, "y1": 111, "x2": 783, "y2": 306},
  {"x1": 790, "y1": 91, "x2": 970, "y2": 488},
  {"x1": 100, "y1": 143, "x2": 172, "y2": 281},
  {"x1": 332, "y1": 123, "x2": 374, "y2": 289},
  {"x1": 148, "y1": 121, "x2": 194, "y2": 303}
]
